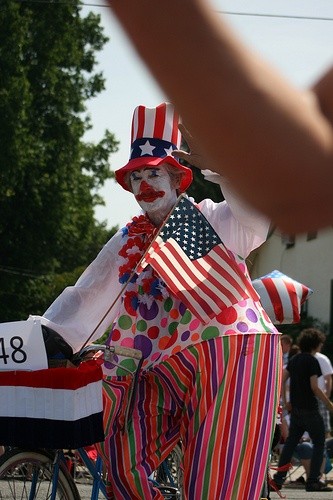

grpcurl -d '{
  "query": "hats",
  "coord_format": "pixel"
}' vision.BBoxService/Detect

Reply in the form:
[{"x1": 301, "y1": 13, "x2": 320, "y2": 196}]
[{"x1": 115, "y1": 101, "x2": 192, "y2": 196}]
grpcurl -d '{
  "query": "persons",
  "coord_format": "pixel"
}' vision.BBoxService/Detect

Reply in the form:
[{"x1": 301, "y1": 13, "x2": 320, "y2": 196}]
[
  {"x1": 100, "y1": 0, "x2": 333, "y2": 234},
  {"x1": 265, "y1": 328, "x2": 333, "y2": 492},
  {"x1": 23, "y1": 100, "x2": 285, "y2": 499}
]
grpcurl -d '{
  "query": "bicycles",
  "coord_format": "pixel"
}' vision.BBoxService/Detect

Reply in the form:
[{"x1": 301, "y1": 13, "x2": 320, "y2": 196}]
[{"x1": 0, "y1": 343, "x2": 185, "y2": 500}]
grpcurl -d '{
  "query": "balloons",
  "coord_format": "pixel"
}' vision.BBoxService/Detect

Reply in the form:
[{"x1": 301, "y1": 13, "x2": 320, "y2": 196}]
[{"x1": 251, "y1": 268, "x2": 314, "y2": 324}]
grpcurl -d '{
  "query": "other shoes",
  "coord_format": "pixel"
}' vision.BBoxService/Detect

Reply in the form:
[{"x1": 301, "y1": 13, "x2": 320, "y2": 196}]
[
  {"x1": 305, "y1": 482, "x2": 332, "y2": 492},
  {"x1": 269, "y1": 474, "x2": 283, "y2": 491}
]
